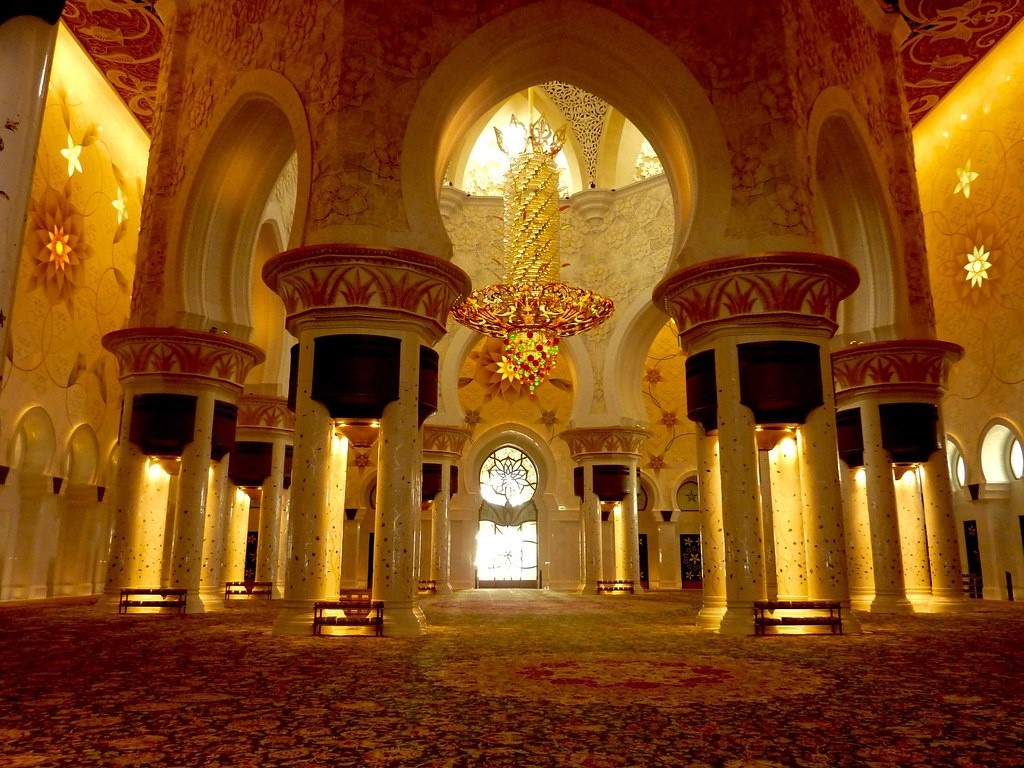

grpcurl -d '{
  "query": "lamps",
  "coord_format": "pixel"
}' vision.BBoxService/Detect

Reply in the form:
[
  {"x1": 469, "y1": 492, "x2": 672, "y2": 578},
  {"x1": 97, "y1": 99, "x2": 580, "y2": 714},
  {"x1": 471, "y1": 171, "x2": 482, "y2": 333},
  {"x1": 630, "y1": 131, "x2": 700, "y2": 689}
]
[{"x1": 448, "y1": 84, "x2": 615, "y2": 401}]
